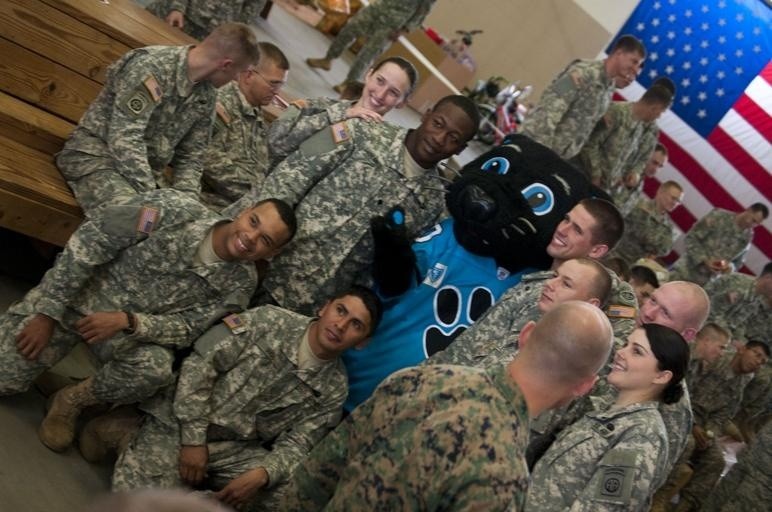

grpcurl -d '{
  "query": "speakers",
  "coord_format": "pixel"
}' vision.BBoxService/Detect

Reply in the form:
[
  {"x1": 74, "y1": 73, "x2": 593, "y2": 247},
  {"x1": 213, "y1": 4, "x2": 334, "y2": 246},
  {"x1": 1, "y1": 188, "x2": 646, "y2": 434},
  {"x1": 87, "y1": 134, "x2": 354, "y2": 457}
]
[
  {"x1": 39, "y1": 375, "x2": 97, "y2": 453},
  {"x1": 81, "y1": 410, "x2": 142, "y2": 463},
  {"x1": 334, "y1": 78, "x2": 353, "y2": 93},
  {"x1": 307, "y1": 56, "x2": 332, "y2": 70},
  {"x1": 651, "y1": 463, "x2": 694, "y2": 511},
  {"x1": 675, "y1": 497, "x2": 693, "y2": 510},
  {"x1": 33, "y1": 371, "x2": 112, "y2": 421},
  {"x1": 736, "y1": 416, "x2": 756, "y2": 442}
]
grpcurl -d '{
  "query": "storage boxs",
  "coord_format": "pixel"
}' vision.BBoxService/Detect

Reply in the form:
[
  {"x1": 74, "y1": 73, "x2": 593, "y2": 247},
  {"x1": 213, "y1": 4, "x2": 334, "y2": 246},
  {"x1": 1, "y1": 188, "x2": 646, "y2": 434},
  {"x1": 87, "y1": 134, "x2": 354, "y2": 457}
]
[{"x1": 0, "y1": 2, "x2": 297, "y2": 250}]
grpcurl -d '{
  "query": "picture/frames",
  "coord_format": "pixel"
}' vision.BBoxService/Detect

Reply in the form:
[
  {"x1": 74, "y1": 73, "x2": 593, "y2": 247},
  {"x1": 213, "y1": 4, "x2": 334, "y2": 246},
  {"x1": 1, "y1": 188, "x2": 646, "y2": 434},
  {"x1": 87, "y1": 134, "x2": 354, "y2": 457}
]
[{"x1": 372, "y1": 22, "x2": 473, "y2": 114}]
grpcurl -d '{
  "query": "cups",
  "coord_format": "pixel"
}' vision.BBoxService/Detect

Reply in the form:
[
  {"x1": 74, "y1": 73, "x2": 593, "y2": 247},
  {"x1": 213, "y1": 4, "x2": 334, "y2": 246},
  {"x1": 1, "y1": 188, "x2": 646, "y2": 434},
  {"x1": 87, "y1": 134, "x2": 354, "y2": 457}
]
[
  {"x1": 748, "y1": 347, "x2": 766, "y2": 365},
  {"x1": 242, "y1": 68, "x2": 282, "y2": 91}
]
